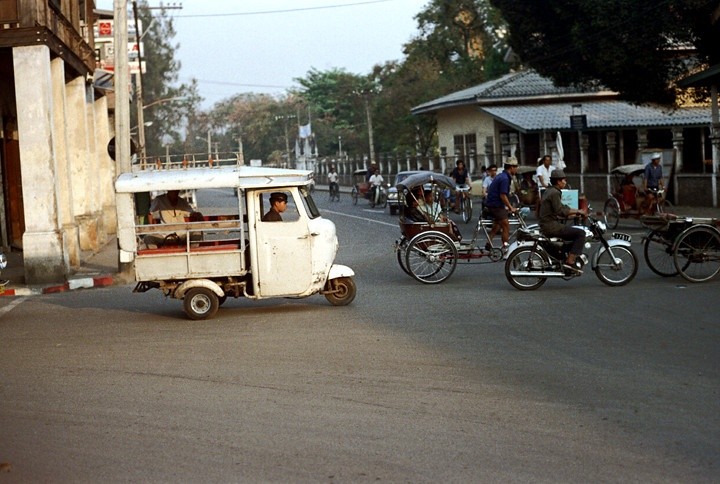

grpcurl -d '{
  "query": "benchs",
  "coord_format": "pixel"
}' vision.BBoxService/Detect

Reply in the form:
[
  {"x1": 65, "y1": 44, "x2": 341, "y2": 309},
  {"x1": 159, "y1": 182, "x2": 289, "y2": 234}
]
[
  {"x1": 137, "y1": 238, "x2": 249, "y2": 255},
  {"x1": 360, "y1": 188, "x2": 368, "y2": 192}
]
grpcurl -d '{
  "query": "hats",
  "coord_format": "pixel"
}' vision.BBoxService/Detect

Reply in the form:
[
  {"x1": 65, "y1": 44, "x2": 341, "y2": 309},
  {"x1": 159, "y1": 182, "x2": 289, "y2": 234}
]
[
  {"x1": 537, "y1": 157, "x2": 543, "y2": 166},
  {"x1": 549, "y1": 169, "x2": 567, "y2": 178},
  {"x1": 271, "y1": 193, "x2": 288, "y2": 203},
  {"x1": 651, "y1": 153, "x2": 661, "y2": 160},
  {"x1": 503, "y1": 157, "x2": 519, "y2": 165}
]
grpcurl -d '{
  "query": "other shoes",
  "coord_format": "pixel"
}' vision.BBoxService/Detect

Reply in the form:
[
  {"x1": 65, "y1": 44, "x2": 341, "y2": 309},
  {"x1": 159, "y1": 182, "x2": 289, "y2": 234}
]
[
  {"x1": 454, "y1": 207, "x2": 459, "y2": 214},
  {"x1": 563, "y1": 261, "x2": 584, "y2": 274},
  {"x1": 485, "y1": 243, "x2": 492, "y2": 251}
]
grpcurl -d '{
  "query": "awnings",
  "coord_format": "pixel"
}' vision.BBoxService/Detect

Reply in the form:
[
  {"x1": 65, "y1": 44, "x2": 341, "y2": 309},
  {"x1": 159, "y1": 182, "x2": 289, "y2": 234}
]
[{"x1": 477, "y1": 100, "x2": 720, "y2": 134}]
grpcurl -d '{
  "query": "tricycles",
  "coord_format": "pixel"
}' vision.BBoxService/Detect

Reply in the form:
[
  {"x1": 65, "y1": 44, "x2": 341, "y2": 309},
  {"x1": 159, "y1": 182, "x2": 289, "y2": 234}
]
[
  {"x1": 641, "y1": 208, "x2": 720, "y2": 284},
  {"x1": 600, "y1": 163, "x2": 679, "y2": 229},
  {"x1": 394, "y1": 170, "x2": 541, "y2": 285},
  {"x1": 447, "y1": 171, "x2": 601, "y2": 240},
  {"x1": 350, "y1": 170, "x2": 386, "y2": 208}
]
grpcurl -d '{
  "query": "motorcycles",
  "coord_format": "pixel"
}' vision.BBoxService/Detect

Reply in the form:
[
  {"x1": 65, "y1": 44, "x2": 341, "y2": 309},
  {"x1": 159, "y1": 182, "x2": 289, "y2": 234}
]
[
  {"x1": 111, "y1": 163, "x2": 358, "y2": 319},
  {"x1": 502, "y1": 201, "x2": 640, "y2": 292}
]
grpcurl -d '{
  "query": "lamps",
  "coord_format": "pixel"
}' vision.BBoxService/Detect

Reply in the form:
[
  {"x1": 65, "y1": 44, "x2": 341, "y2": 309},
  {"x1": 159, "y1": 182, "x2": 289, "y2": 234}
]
[{"x1": 86, "y1": 68, "x2": 115, "y2": 92}]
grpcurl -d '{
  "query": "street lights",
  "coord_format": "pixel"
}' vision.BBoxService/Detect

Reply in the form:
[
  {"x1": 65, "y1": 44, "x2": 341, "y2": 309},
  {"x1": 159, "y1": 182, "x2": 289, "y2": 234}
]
[{"x1": 138, "y1": 96, "x2": 190, "y2": 168}]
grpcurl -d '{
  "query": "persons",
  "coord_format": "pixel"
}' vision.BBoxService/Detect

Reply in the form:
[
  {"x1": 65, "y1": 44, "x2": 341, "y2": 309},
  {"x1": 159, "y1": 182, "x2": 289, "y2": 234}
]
[
  {"x1": 536, "y1": 155, "x2": 556, "y2": 200},
  {"x1": 522, "y1": 173, "x2": 536, "y2": 205},
  {"x1": 642, "y1": 153, "x2": 665, "y2": 216},
  {"x1": 144, "y1": 190, "x2": 203, "y2": 250},
  {"x1": 328, "y1": 168, "x2": 339, "y2": 197},
  {"x1": 484, "y1": 157, "x2": 518, "y2": 253},
  {"x1": 619, "y1": 173, "x2": 642, "y2": 210},
  {"x1": 369, "y1": 168, "x2": 388, "y2": 205},
  {"x1": 449, "y1": 159, "x2": 472, "y2": 214},
  {"x1": 261, "y1": 192, "x2": 288, "y2": 222},
  {"x1": 537, "y1": 168, "x2": 588, "y2": 273},
  {"x1": 404, "y1": 185, "x2": 463, "y2": 242},
  {"x1": 481, "y1": 164, "x2": 497, "y2": 218}
]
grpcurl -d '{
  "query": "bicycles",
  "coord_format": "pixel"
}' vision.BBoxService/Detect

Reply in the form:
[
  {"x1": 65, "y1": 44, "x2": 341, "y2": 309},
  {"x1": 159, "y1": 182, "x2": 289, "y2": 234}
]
[{"x1": 329, "y1": 181, "x2": 341, "y2": 202}]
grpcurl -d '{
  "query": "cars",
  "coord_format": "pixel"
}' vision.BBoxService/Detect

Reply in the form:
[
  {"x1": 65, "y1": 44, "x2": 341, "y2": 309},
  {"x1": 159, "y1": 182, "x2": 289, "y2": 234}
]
[{"x1": 386, "y1": 171, "x2": 438, "y2": 215}]
